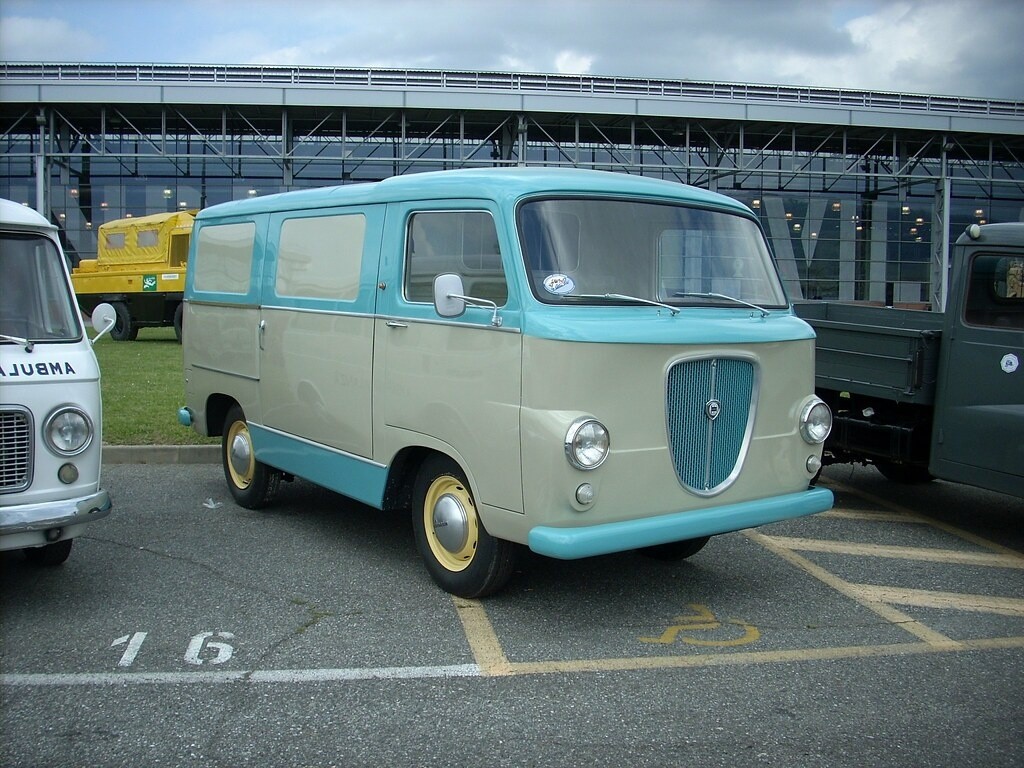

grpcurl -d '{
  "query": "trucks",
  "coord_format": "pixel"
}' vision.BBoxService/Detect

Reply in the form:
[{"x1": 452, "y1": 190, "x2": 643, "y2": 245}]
[
  {"x1": 796, "y1": 222, "x2": 1024, "y2": 521},
  {"x1": 67, "y1": 211, "x2": 195, "y2": 342}
]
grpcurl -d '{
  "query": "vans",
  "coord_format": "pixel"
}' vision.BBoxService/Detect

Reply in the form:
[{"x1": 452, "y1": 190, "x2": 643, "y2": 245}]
[{"x1": 0, "y1": 199, "x2": 123, "y2": 571}]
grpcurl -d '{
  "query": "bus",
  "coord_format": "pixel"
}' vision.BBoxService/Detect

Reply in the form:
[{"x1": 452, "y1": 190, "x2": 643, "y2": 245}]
[{"x1": 178, "y1": 168, "x2": 836, "y2": 602}]
